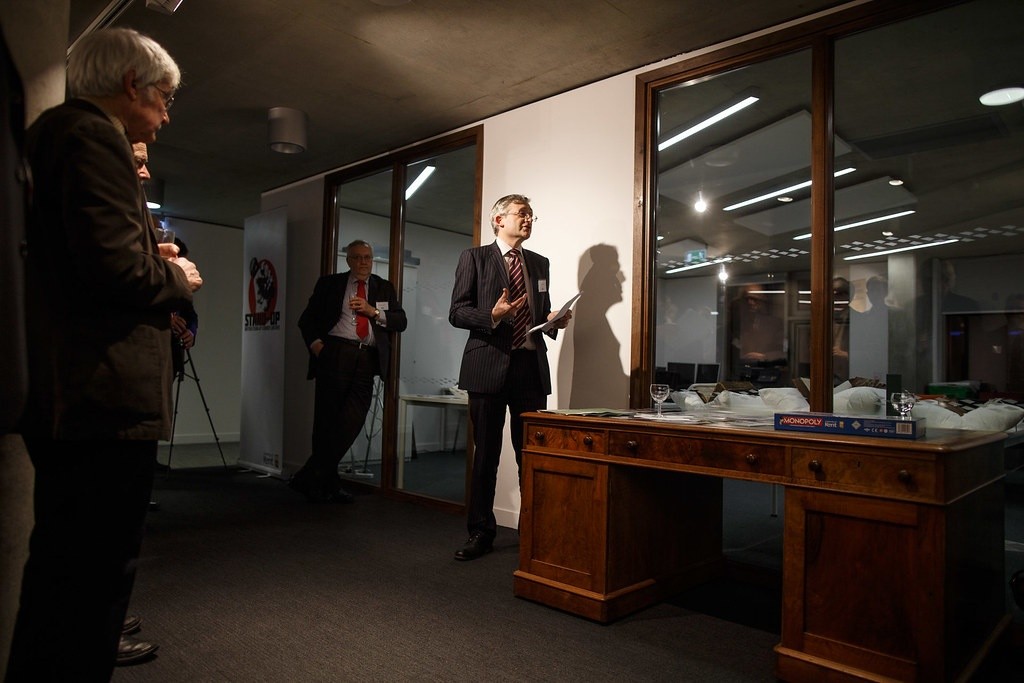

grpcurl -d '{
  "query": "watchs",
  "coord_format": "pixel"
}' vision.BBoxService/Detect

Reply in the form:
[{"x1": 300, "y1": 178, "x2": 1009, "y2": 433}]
[{"x1": 371, "y1": 309, "x2": 380, "y2": 319}]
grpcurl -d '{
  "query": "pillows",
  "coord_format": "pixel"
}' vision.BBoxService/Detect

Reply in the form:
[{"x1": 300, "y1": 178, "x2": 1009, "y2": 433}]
[{"x1": 669, "y1": 380, "x2": 1024, "y2": 431}]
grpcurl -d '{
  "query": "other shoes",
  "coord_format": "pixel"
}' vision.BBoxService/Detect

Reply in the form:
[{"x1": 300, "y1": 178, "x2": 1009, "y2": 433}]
[
  {"x1": 297, "y1": 472, "x2": 354, "y2": 505},
  {"x1": 119, "y1": 605, "x2": 143, "y2": 634},
  {"x1": 115, "y1": 634, "x2": 160, "y2": 662}
]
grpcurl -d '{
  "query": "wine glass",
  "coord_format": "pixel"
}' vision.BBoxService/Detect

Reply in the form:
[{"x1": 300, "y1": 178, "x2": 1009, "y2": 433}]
[
  {"x1": 891, "y1": 393, "x2": 915, "y2": 417},
  {"x1": 349, "y1": 292, "x2": 360, "y2": 325},
  {"x1": 650, "y1": 383, "x2": 670, "y2": 418}
]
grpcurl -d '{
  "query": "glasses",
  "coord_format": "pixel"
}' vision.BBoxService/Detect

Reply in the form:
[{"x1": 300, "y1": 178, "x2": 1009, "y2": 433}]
[
  {"x1": 500, "y1": 210, "x2": 538, "y2": 223},
  {"x1": 348, "y1": 254, "x2": 373, "y2": 263},
  {"x1": 151, "y1": 84, "x2": 175, "y2": 111},
  {"x1": 134, "y1": 157, "x2": 149, "y2": 169}
]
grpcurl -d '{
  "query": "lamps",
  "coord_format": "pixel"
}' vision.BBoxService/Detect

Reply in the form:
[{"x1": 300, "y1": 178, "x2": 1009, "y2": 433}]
[
  {"x1": 267, "y1": 108, "x2": 308, "y2": 155},
  {"x1": 142, "y1": 177, "x2": 165, "y2": 209},
  {"x1": 793, "y1": 208, "x2": 916, "y2": 241},
  {"x1": 721, "y1": 161, "x2": 856, "y2": 211},
  {"x1": 655, "y1": 86, "x2": 760, "y2": 151}
]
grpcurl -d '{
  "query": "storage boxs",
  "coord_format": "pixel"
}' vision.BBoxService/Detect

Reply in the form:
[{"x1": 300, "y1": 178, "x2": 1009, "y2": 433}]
[{"x1": 774, "y1": 412, "x2": 926, "y2": 440}]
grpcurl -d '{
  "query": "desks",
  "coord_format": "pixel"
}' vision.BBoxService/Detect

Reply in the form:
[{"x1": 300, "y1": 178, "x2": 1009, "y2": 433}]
[
  {"x1": 514, "y1": 409, "x2": 1012, "y2": 683},
  {"x1": 399, "y1": 395, "x2": 469, "y2": 489}
]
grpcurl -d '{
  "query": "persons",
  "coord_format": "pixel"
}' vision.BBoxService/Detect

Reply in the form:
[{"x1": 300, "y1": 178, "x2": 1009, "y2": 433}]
[
  {"x1": 0, "y1": 28, "x2": 202, "y2": 680},
  {"x1": 292, "y1": 240, "x2": 407, "y2": 503},
  {"x1": 726, "y1": 259, "x2": 1024, "y2": 401},
  {"x1": 448, "y1": 195, "x2": 572, "y2": 561}
]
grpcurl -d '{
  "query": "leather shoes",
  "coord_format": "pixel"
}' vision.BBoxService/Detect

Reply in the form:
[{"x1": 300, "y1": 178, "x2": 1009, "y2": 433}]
[{"x1": 455, "y1": 528, "x2": 493, "y2": 561}]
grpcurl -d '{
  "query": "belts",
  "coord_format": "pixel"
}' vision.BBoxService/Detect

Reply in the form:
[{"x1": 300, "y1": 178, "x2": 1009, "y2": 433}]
[
  {"x1": 510, "y1": 349, "x2": 538, "y2": 360},
  {"x1": 323, "y1": 334, "x2": 379, "y2": 354}
]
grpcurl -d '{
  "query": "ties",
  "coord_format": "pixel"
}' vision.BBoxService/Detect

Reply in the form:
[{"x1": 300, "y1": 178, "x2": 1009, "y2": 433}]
[
  {"x1": 507, "y1": 250, "x2": 533, "y2": 350},
  {"x1": 356, "y1": 280, "x2": 369, "y2": 341}
]
